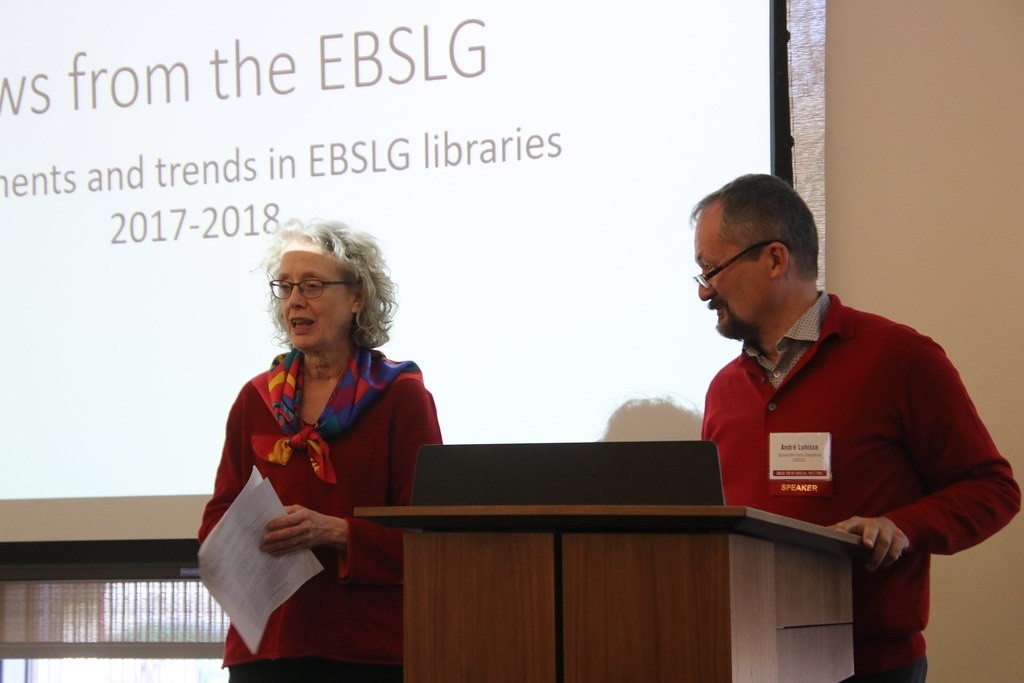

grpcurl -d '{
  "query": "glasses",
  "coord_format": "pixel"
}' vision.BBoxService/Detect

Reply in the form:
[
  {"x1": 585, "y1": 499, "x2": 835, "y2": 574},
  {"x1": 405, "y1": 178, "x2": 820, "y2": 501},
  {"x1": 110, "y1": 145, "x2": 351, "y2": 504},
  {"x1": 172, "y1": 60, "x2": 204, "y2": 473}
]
[
  {"x1": 268, "y1": 275, "x2": 355, "y2": 299},
  {"x1": 693, "y1": 239, "x2": 791, "y2": 289}
]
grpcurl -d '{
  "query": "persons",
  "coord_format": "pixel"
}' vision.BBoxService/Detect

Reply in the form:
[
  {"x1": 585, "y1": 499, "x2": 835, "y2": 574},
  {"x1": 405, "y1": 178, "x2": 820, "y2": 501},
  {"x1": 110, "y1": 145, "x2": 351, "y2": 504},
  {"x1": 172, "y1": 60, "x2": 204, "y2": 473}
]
[
  {"x1": 197, "y1": 224, "x2": 444, "y2": 683},
  {"x1": 688, "y1": 173, "x2": 1021, "y2": 683}
]
